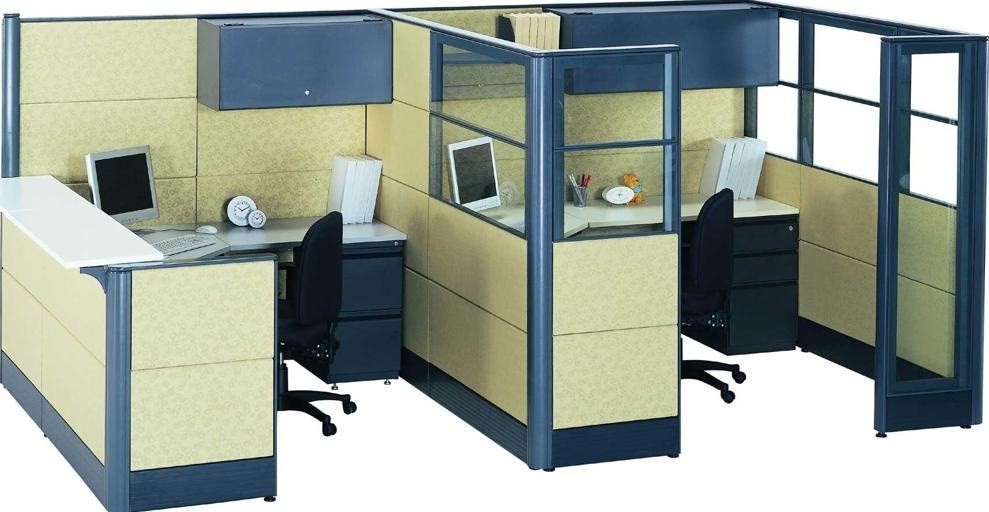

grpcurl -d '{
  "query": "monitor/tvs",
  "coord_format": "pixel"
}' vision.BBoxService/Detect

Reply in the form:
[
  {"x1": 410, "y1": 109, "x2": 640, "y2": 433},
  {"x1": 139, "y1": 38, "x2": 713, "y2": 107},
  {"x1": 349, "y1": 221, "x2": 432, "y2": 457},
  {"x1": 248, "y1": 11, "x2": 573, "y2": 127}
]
[
  {"x1": 85, "y1": 145, "x2": 159, "y2": 232},
  {"x1": 444, "y1": 135, "x2": 501, "y2": 212}
]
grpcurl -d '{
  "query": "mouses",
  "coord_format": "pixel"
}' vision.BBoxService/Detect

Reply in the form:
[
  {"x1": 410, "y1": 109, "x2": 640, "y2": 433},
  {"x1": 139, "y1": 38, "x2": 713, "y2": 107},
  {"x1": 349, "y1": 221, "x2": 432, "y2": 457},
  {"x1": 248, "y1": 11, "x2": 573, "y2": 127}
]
[{"x1": 195, "y1": 225, "x2": 218, "y2": 234}]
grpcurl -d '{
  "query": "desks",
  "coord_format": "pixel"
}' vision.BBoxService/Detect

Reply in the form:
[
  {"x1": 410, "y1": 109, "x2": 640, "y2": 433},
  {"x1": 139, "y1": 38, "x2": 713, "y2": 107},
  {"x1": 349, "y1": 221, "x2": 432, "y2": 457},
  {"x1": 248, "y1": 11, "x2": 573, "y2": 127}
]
[
  {"x1": 132, "y1": 216, "x2": 407, "y2": 384},
  {"x1": 471, "y1": 192, "x2": 797, "y2": 356}
]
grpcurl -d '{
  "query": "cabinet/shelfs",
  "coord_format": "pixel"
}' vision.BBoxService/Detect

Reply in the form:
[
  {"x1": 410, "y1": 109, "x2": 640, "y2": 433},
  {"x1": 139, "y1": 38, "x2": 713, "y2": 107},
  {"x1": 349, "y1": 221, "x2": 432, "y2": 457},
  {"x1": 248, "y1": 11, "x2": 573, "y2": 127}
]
[
  {"x1": 197, "y1": 14, "x2": 394, "y2": 112},
  {"x1": 543, "y1": 4, "x2": 778, "y2": 95}
]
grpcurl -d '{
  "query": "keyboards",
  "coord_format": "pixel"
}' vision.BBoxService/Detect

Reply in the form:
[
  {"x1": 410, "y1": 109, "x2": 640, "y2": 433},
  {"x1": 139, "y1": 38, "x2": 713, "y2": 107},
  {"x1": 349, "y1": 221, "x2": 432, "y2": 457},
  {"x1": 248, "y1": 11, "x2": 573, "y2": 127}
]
[{"x1": 150, "y1": 234, "x2": 216, "y2": 256}]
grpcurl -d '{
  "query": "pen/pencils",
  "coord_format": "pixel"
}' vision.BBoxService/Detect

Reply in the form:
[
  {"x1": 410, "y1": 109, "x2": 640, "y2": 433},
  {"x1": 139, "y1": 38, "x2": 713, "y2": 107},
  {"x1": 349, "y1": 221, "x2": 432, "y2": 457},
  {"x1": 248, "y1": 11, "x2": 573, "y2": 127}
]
[{"x1": 567, "y1": 173, "x2": 591, "y2": 206}]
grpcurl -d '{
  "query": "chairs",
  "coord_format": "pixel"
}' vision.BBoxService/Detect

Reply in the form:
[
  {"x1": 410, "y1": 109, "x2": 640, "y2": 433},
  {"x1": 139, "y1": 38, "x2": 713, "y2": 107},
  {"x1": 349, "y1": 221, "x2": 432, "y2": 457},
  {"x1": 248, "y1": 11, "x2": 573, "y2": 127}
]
[
  {"x1": 275, "y1": 210, "x2": 357, "y2": 434},
  {"x1": 681, "y1": 188, "x2": 745, "y2": 403}
]
